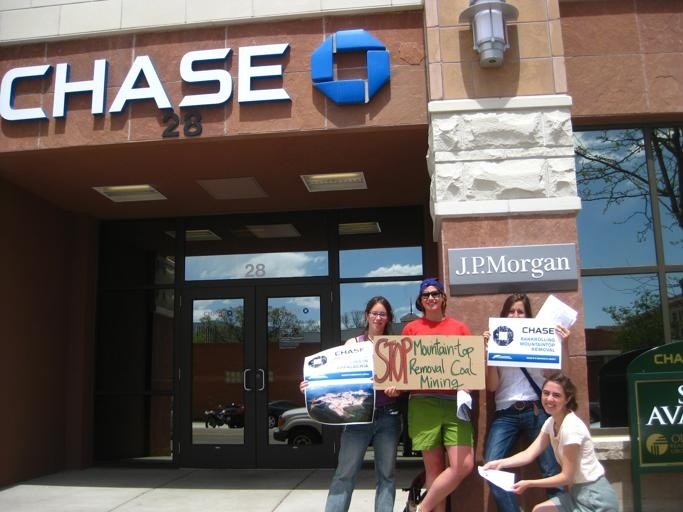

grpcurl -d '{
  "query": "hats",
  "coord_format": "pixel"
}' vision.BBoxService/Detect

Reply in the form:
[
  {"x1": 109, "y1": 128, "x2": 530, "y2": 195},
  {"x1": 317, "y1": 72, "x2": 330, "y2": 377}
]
[{"x1": 416, "y1": 279, "x2": 443, "y2": 311}]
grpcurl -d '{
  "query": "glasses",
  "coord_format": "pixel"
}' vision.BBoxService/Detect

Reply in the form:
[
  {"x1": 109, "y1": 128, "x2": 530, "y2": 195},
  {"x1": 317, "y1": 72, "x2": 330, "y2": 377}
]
[{"x1": 421, "y1": 291, "x2": 440, "y2": 299}]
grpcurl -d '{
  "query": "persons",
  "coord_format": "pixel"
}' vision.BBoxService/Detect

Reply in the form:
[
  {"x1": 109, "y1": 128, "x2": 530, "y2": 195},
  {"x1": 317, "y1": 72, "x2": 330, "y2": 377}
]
[
  {"x1": 481, "y1": 292, "x2": 573, "y2": 512},
  {"x1": 483, "y1": 371, "x2": 620, "y2": 512},
  {"x1": 383, "y1": 278, "x2": 475, "y2": 512},
  {"x1": 299, "y1": 295, "x2": 408, "y2": 512}
]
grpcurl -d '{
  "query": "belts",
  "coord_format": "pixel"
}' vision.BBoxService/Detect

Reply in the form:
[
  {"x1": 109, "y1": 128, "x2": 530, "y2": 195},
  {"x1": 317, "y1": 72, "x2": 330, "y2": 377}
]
[{"x1": 513, "y1": 401, "x2": 536, "y2": 411}]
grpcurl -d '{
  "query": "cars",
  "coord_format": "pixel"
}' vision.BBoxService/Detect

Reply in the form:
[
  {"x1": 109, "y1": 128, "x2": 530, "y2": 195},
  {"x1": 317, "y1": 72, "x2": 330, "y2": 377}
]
[{"x1": 227, "y1": 400, "x2": 322, "y2": 446}]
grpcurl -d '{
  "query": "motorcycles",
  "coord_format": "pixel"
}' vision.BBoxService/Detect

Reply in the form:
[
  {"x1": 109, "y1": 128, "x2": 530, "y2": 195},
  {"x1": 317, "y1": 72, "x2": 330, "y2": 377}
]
[{"x1": 204, "y1": 408, "x2": 227, "y2": 428}]
